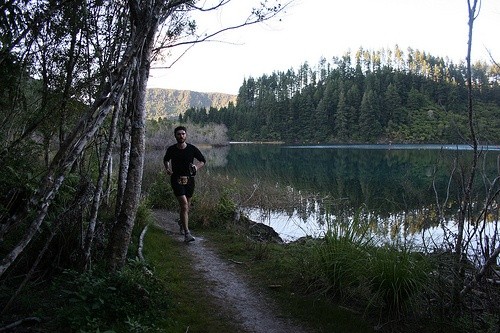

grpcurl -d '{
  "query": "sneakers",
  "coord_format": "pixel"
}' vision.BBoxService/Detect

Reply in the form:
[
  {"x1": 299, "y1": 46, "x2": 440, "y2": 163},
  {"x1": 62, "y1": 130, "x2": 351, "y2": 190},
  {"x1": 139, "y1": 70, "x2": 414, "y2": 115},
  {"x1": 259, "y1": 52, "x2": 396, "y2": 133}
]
[
  {"x1": 178, "y1": 219, "x2": 185, "y2": 234},
  {"x1": 184, "y1": 229, "x2": 195, "y2": 243}
]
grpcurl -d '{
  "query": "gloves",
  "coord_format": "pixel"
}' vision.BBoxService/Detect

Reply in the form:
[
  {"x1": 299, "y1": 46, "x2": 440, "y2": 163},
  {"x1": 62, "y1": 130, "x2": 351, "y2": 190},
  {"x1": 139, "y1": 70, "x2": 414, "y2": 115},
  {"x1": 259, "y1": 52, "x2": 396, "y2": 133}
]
[{"x1": 189, "y1": 164, "x2": 198, "y2": 177}]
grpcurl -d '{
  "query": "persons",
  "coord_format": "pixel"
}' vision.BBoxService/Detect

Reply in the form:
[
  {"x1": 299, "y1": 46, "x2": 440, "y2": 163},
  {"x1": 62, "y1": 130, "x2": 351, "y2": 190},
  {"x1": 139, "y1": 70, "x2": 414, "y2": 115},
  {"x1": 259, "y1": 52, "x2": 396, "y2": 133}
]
[{"x1": 163, "y1": 126, "x2": 206, "y2": 244}]
